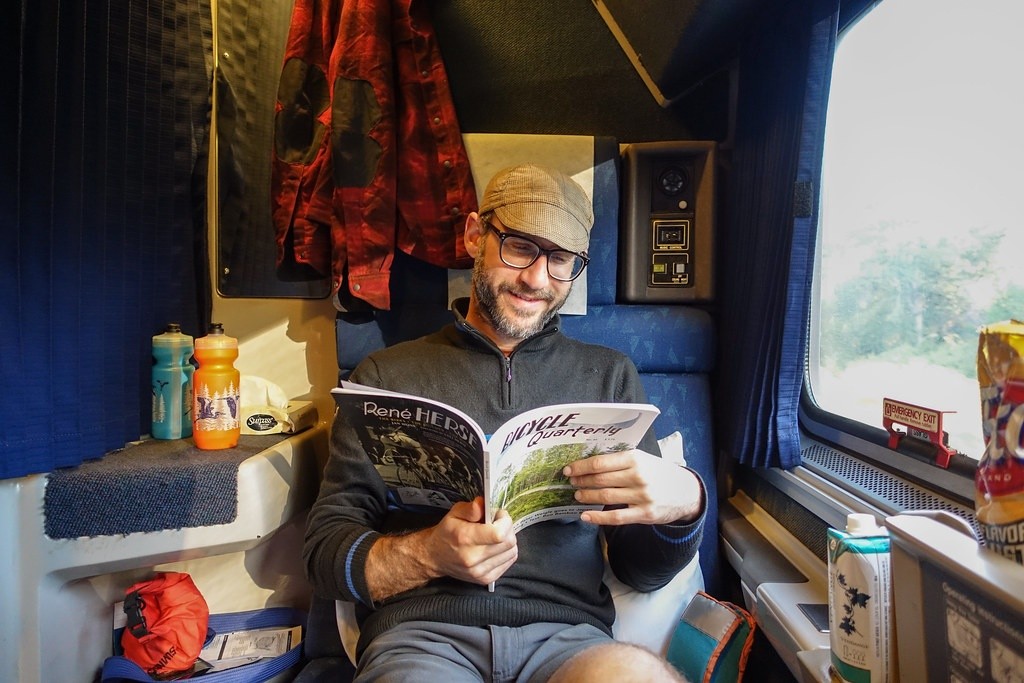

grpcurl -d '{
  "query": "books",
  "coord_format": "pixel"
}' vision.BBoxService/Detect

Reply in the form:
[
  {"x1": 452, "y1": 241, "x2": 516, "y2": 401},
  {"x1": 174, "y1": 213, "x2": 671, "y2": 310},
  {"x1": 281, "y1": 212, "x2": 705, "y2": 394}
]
[{"x1": 330, "y1": 379, "x2": 662, "y2": 592}]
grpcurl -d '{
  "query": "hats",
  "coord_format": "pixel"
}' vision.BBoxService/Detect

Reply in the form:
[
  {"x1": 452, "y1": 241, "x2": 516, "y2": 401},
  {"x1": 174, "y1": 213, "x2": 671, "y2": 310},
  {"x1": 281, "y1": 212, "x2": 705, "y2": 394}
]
[{"x1": 478, "y1": 163, "x2": 595, "y2": 251}]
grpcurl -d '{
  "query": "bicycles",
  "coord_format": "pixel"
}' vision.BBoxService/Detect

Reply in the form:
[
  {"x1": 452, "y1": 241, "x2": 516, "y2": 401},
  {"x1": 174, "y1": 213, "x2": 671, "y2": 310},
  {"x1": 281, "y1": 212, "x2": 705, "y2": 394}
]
[{"x1": 392, "y1": 455, "x2": 476, "y2": 502}]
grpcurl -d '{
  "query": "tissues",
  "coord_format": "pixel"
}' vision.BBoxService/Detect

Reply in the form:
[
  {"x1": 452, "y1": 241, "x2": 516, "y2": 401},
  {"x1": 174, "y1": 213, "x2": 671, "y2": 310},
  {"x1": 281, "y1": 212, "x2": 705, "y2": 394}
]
[{"x1": 240, "y1": 376, "x2": 318, "y2": 435}]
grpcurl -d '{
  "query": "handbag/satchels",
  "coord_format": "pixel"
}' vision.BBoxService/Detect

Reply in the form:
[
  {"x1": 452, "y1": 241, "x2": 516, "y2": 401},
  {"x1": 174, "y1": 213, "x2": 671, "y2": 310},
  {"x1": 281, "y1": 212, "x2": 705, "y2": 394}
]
[{"x1": 121, "y1": 572, "x2": 209, "y2": 678}]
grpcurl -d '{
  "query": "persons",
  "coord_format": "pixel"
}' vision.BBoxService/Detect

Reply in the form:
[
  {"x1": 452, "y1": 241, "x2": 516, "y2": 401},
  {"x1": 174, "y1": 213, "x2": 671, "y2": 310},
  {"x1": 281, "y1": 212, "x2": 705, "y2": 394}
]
[{"x1": 303, "y1": 164, "x2": 709, "y2": 683}]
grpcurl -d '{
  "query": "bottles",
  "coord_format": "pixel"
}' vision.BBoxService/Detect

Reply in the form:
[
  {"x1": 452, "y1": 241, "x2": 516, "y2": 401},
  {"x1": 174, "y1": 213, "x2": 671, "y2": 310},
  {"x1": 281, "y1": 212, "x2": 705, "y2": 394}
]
[
  {"x1": 152, "y1": 323, "x2": 194, "y2": 440},
  {"x1": 192, "y1": 323, "x2": 240, "y2": 451}
]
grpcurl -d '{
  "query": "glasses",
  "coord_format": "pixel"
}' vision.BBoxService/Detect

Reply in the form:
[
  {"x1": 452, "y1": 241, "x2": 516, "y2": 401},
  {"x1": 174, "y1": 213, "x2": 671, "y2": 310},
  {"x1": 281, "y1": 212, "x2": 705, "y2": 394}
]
[{"x1": 484, "y1": 220, "x2": 590, "y2": 282}]
